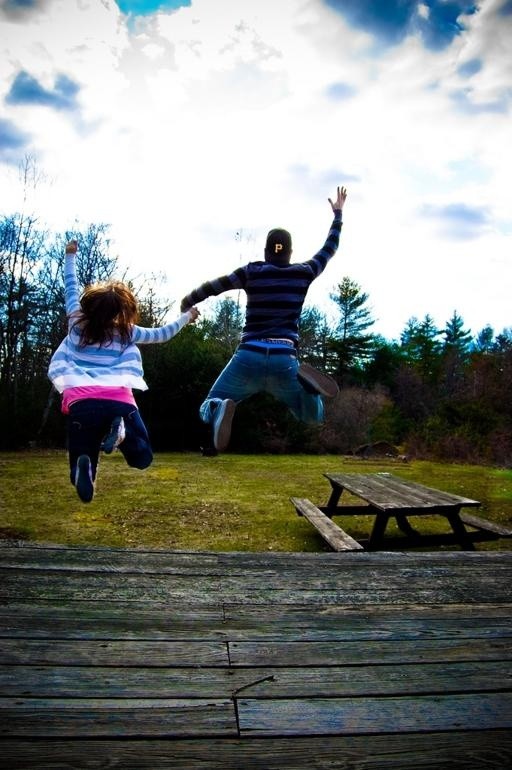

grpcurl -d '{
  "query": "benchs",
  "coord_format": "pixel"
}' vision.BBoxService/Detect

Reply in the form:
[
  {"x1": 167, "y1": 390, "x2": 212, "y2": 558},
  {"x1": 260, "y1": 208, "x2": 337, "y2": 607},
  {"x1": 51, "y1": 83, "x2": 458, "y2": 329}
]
[
  {"x1": 288, "y1": 497, "x2": 365, "y2": 552},
  {"x1": 458, "y1": 512, "x2": 511, "y2": 540}
]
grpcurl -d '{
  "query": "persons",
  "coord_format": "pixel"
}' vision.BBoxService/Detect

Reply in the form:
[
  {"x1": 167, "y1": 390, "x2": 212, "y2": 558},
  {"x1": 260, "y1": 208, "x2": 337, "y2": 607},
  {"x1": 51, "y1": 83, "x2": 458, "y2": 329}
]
[
  {"x1": 180, "y1": 185, "x2": 348, "y2": 451},
  {"x1": 47, "y1": 239, "x2": 201, "y2": 504}
]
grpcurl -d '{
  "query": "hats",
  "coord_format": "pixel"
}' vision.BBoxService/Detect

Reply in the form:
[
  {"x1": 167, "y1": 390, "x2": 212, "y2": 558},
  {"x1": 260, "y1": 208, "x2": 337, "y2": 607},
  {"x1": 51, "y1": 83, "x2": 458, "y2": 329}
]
[{"x1": 268, "y1": 228, "x2": 291, "y2": 265}]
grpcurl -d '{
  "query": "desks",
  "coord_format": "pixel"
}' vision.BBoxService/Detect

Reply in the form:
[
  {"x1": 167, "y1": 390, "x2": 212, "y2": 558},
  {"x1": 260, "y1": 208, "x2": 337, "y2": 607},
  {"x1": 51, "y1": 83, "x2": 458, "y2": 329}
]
[{"x1": 322, "y1": 473, "x2": 482, "y2": 552}]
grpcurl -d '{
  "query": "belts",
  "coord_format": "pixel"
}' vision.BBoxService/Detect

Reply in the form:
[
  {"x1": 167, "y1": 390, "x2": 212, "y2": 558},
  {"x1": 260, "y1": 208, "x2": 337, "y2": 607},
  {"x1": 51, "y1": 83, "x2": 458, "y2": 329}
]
[{"x1": 239, "y1": 344, "x2": 297, "y2": 356}]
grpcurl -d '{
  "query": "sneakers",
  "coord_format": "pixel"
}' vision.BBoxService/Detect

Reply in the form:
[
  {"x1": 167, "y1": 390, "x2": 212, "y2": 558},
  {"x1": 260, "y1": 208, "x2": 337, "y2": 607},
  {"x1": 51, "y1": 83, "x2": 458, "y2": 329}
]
[
  {"x1": 75, "y1": 455, "x2": 93, "y2": 503},
  {"x1": 104, "y1": 415, "x2": 125, "y2": 454},
  {"x1": 298, "y1": 362, "x2": 340, "y2": 398},
  {"x1": 211, "y1": 398, "x2": 236, "y2": 450}
]
